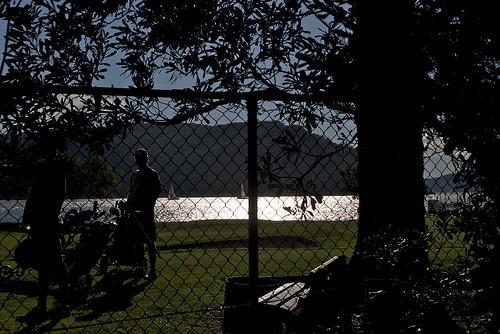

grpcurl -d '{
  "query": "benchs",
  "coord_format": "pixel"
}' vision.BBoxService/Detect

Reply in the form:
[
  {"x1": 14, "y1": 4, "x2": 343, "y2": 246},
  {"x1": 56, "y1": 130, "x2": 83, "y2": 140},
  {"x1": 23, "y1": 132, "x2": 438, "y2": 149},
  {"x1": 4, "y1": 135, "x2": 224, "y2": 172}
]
[{"x1": 258, "y1": 253, "x2": 347, "y2": 330}]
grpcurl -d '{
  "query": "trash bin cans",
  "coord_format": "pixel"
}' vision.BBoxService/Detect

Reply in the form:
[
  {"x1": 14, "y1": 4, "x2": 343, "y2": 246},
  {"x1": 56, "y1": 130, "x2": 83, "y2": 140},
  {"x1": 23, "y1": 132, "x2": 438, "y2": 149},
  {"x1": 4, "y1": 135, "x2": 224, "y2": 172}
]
[{"x1": 222, "y1": 275, "x2": 282, "y2": 334}]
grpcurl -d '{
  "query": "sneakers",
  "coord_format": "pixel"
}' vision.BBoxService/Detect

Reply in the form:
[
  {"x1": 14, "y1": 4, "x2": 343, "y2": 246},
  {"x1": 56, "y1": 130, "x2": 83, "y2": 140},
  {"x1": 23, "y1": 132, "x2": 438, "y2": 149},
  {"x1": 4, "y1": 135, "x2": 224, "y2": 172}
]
[
  {"x1": 48, "y1": 305, "x2": 70, "y2": 320},
  {"x1": 16, "y1": 304, "x2": 48, "y2": 323},
  {"x1": 133, "y1": 268, "x2": 148, "y2": 275},
  {"x1": 143, "y1": 270, "x2": 157, "y2": 280}
]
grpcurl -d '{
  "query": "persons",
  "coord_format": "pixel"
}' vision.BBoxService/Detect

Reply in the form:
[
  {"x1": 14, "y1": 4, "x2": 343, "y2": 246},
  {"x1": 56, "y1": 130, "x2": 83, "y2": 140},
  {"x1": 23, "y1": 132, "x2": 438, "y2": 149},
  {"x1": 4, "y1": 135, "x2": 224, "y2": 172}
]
[
  {"x1": 16, "y1": 140, "x2": 71, "y2": 323},
  {"x1": 125, "y1": 147, "x2": 162, "y2": 281}
]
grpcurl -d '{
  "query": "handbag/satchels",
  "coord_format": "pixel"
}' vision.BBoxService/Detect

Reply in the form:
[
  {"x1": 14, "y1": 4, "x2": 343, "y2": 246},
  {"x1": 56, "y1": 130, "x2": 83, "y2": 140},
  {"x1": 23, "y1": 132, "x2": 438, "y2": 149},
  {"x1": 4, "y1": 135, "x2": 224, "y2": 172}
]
[{"x1": 14, "y1": 231, "x2": 55, "y2": 274}]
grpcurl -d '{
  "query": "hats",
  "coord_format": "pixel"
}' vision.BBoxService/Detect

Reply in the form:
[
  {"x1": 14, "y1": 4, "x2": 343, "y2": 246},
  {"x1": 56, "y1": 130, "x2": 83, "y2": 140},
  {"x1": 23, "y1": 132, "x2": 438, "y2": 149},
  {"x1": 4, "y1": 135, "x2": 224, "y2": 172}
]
[{"x1": 133, "y1": 148, "x2": 148, "y2": 157}]
